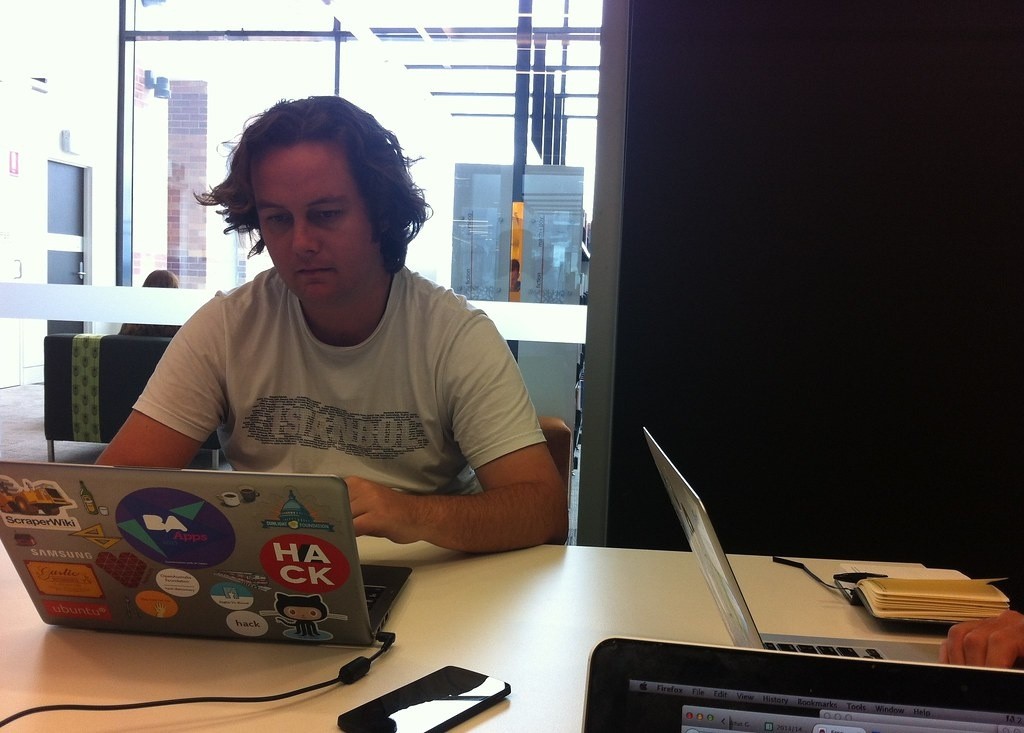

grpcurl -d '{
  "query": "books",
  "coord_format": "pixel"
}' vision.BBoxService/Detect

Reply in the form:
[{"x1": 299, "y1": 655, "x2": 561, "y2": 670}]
[{"x1": 834, "y1": 576, "x2": 1010, "y2": 628}]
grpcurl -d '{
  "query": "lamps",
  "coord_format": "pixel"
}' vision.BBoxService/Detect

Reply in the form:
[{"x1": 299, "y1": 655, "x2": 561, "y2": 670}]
[{"x1": 145, "y1": 70, "x2": 171, "y2": 100}]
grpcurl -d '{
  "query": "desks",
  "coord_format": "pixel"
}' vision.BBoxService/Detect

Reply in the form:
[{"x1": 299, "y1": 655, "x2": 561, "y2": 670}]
[{"x1": 0, "y1": 534, "x2": 972, "y2": 733}]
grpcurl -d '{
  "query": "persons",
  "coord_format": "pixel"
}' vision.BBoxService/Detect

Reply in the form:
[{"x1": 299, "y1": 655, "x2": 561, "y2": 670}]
[
  {"x1": 939, "y1": 608, "x2": 1024, "y2": 668},
  {"x1": 117, "y1": 270, "x2": 182, "y2": 338},
  {"x1": 96, "y1": 97, "x2": 569, "y2": 554}
]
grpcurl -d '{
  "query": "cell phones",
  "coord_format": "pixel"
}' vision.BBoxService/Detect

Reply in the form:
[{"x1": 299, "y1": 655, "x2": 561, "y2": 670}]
[{"x1": 338, "y1": 665, "x2": 512, "y2": 733}]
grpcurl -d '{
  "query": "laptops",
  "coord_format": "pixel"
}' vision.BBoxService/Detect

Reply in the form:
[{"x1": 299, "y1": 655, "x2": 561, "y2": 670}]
[
  {"x1": 581, "y1": 634, "x2": 1024, "y2": 733},
  {"x1": 642, "y1": 426, "x2": 944, "y2": 664},
  {"x1": 0, "y1": 459, "x2": 412, "y2": 646}
]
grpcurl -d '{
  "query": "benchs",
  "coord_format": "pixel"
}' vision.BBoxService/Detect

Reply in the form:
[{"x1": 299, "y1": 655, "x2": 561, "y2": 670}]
[{"x1": 42, "y1": 333, "x2": 223, "y2": 470}]
